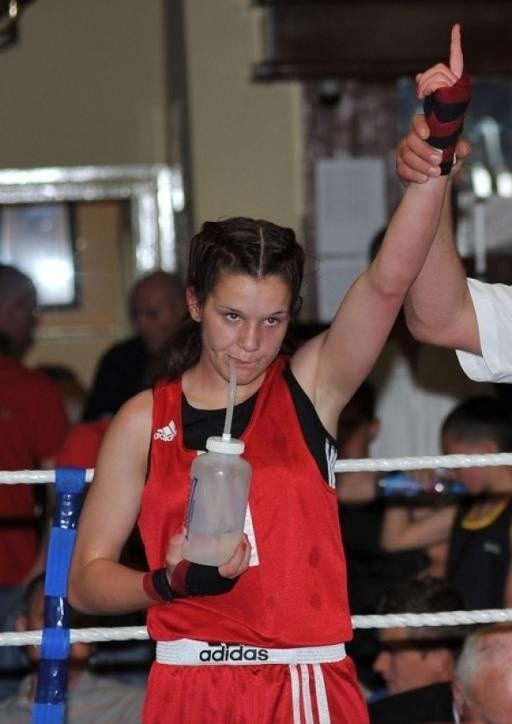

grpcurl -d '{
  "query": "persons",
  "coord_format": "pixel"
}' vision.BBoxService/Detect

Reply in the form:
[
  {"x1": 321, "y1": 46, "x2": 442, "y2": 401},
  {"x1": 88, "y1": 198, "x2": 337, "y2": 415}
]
[
  {"x1": 332, "y1": 224, "x2": 512, "y2": 724},
  {"x1": 389, "y1": 112, "x2": 509, "y2": 385},
  {"x1": 64, "y1": 21, "x2": 476, "y2": 724},
  {"x1": 0, "y1": 266, "x2": 188, "y2": 724}
]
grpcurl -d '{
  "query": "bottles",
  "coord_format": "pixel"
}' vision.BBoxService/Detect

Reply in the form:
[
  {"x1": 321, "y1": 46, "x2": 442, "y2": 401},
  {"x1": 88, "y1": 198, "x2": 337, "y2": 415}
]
[{"x1": 372, "y1": 469, "x2": 463, "y2": 500}]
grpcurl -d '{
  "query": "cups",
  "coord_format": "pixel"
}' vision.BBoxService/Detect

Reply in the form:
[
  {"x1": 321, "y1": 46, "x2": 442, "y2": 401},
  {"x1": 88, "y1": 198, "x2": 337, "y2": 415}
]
[{"x1": 181, "y1": 433, "x2": 252, "y2": 567}]
